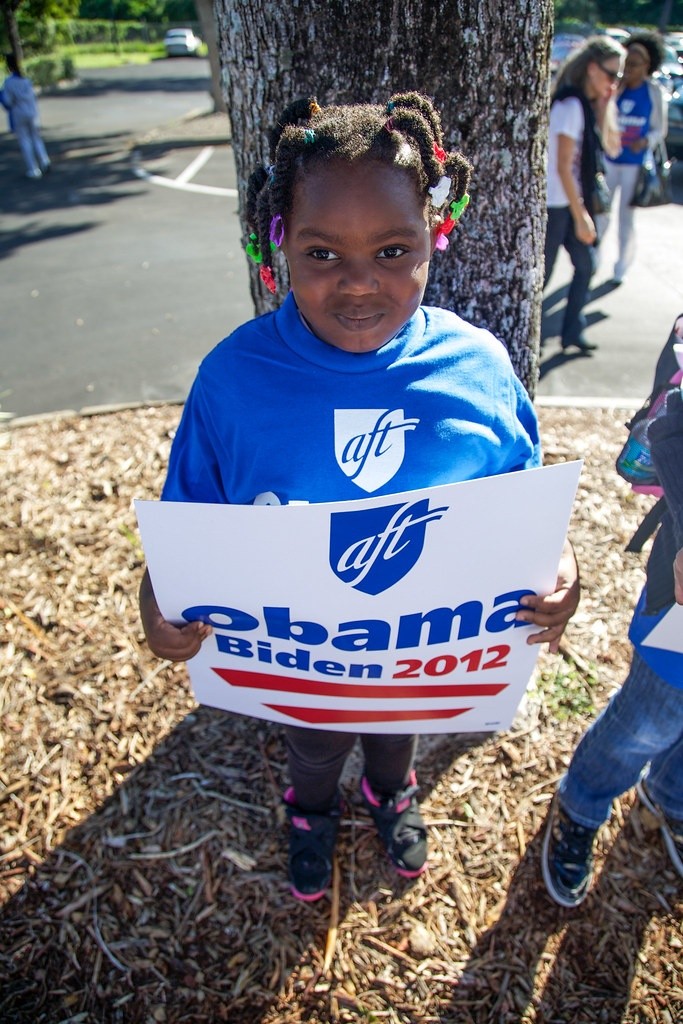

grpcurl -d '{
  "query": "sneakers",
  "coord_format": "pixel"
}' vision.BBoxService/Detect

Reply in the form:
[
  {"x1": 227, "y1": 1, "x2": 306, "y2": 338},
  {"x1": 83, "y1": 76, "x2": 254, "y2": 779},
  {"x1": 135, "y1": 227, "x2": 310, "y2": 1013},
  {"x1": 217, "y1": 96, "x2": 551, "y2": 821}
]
[
  {"x1": 636, "y1": 767, "x2": 683, "y2": 879},
  {"x1": 280, "y1": 785, "x2": 343, "y2": 900},
  {"x1": 541, "y1": 775, "x2": 600, "y2": 908},
  {"x1": 358, "y1": 765, "x2": 429, "y2": 878}
]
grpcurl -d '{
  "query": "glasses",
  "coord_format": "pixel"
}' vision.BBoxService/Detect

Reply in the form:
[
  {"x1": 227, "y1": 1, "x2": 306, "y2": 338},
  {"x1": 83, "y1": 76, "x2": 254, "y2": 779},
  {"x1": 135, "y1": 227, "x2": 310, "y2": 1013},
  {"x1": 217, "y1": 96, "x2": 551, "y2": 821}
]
[{"x1": 597, "y1": 62, "x2": 624, "y2": 85}]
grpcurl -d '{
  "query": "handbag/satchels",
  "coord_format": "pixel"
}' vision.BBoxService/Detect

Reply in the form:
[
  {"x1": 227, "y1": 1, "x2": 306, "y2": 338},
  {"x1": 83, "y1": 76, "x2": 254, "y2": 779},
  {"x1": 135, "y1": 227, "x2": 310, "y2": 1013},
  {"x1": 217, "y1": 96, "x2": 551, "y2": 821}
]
[
  {"x1": 628, "y1": 140, "x2": 675, "y2": 207},
  {"x1": 590, "y1": 172, "x2": 611, "y2": 216}
]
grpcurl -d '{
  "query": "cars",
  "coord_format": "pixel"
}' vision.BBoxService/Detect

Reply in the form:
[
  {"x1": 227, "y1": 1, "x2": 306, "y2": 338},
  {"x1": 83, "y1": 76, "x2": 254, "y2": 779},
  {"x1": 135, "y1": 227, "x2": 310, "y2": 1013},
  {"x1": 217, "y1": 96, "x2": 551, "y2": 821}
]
[
  {"x1": 547, "y1": 24, "x2": 683, "y2": 170},
  {"x1": 162, "y1": 28, "x2": 199, "y2": 56}
]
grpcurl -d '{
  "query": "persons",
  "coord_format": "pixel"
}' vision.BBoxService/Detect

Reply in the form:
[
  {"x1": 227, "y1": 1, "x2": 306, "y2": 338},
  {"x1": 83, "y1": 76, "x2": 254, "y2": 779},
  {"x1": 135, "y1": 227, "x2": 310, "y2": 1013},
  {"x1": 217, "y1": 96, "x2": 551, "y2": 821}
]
[
  {"x1": 543, "y1": 383, "x2": 683, "y2": 909},
  {"x1": 2, "y1": 52, "x2": 54, "y2": 179},
  {"x1": 141, "y1": 89, "x2": 583, "y2": 904},
  {"x1": 588, "y1": 31, "x2": 669, "y2": 285},
  {"x1": 544, "y1": 38, "x2": 630, "y2": 356}
]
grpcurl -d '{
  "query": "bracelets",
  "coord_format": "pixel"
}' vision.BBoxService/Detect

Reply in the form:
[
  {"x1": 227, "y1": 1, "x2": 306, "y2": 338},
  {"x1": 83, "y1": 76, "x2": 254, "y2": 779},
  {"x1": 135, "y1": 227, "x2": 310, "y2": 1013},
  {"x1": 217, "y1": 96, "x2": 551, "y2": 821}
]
[{"x1": 570, "y1": 199, "x2": 585, "y2": 208}]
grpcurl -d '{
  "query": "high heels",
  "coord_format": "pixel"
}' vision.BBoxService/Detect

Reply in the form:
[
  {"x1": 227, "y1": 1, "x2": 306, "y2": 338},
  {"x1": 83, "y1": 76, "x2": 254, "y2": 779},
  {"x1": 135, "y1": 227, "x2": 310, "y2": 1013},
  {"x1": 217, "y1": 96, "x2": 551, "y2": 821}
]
[{"x1": 562, "y1": 333, "x2": 599, "y2": 354}]
